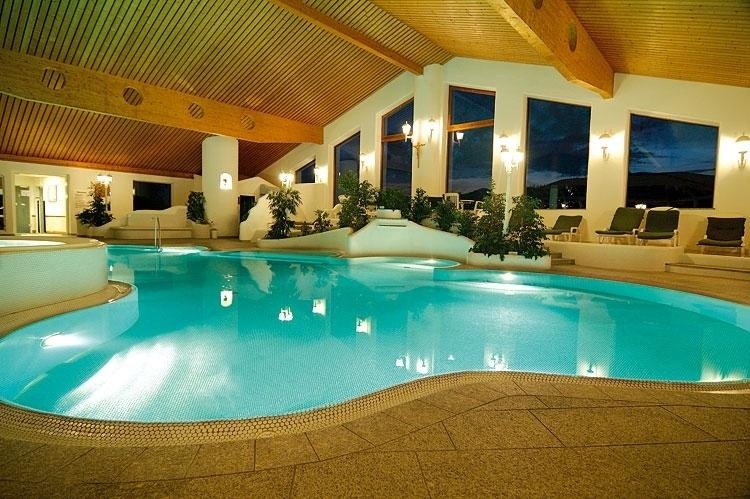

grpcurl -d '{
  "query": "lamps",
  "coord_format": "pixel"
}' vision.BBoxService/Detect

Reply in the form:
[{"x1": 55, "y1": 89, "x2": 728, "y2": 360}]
[
  {"x1": 498, "y1": 132, "x2": 520, "y2": 172},
  {"x1": 402, "y1": 119, "x2": 425, "y2": 167},
  {"x1": 599, "y1": 131, "x2": 611, "y2": 161},
  {"x1": 735, "y1": 132, "x2": 750, "y2": 171},
  {"x1": 448, "y1": 130, "x2": 464, "y2": 147}
]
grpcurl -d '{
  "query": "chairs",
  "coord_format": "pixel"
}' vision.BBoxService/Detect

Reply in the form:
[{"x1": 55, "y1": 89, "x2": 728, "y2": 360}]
[
  {"x1": 636, "y1": 206, "x2": 681, "y2": 247},
  {"x1": 696, "y1": 215, "x2": 746, "y2": 256},
  {"x1": 542, "y1": 215, "x2": 584, "y2": 242},
  {"x1": 595, "y1": 207, "x2": 645, "y2": 244}
]
